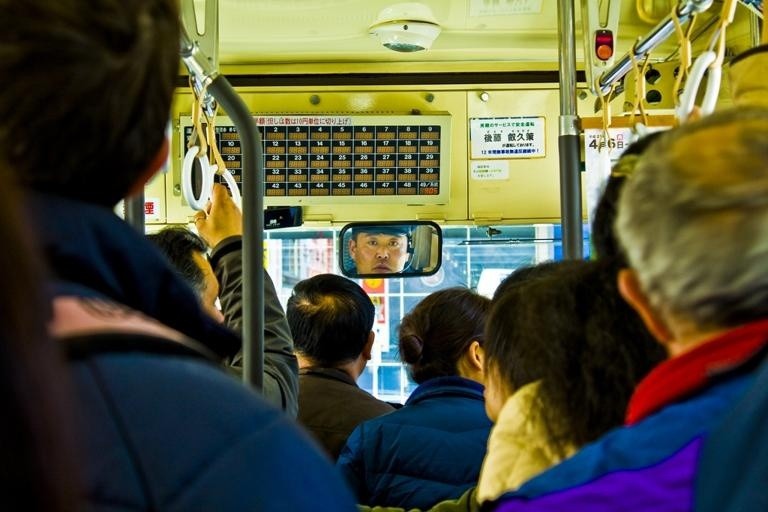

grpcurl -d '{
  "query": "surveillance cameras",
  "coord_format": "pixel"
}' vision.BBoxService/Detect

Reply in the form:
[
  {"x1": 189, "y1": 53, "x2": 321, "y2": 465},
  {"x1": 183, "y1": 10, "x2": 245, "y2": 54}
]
[{"x1": 370, "y1": 3, "x2": 442, "y2": 53}]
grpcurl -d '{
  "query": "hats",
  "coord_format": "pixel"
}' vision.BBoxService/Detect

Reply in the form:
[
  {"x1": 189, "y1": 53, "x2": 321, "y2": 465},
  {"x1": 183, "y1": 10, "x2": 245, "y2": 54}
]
[{"x1": 350, "y1": 223, "x2": 417, "y2": 235}]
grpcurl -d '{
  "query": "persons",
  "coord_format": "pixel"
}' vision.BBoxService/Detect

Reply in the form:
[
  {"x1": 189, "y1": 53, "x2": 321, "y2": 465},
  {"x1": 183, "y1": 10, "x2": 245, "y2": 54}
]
[
  {"x1": 144, "y1": 182, "x2": 302, "y2": 420},
  {"x1": 347, "y1": 224, "x2": 424, "y2": 273},
  {"x1": 0, "y1": 13, "x2": 357, "y2": 511},
  {"x1": 432, "y1": 42, "x2": 768, "y2": 511},
  {"x1": 336, "y1": 286, "x2": 494, "y2": 512},
  {"x1": 285, "y1": 272, "x2": 396, "y2": 465}
]
